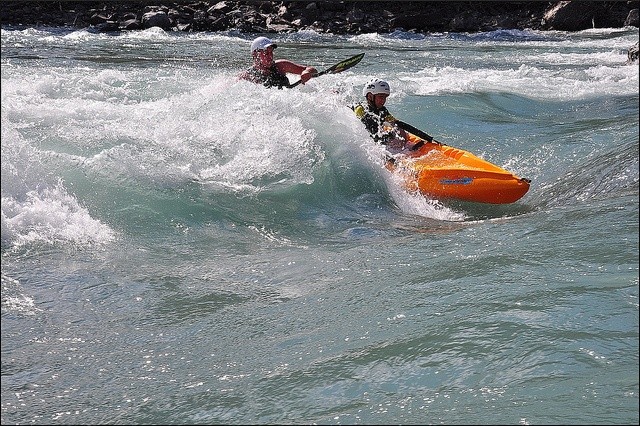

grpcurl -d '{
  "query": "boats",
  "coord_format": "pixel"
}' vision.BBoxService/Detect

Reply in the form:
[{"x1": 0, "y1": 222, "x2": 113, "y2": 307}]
[{"x1": 383, "y1": 126, "x2": 531, "y2": 204}]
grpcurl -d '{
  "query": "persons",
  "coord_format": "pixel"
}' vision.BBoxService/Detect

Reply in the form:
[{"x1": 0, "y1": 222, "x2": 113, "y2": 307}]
[
  {"x1": 342, "y1": 80, "x2": 409, "y2": 162},
  {"x1": 232, "y1": 37, "x2": 319, "y2": 92}
]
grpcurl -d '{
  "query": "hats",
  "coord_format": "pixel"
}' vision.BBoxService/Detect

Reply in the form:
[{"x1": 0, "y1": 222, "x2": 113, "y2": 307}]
[{"x1": 251, "y1": 36, "x2": 278, "y2": 53}]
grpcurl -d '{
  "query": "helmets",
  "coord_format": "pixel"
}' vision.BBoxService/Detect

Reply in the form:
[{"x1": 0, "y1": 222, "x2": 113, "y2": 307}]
[{"x1": 363, "y1": 78, "x2": 389, "y2": 96}]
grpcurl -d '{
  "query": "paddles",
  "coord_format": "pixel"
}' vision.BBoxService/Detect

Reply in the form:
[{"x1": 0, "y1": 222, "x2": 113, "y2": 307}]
[{"x1": 286, "y1": 53, "x2": 365, "y2": 89}]
